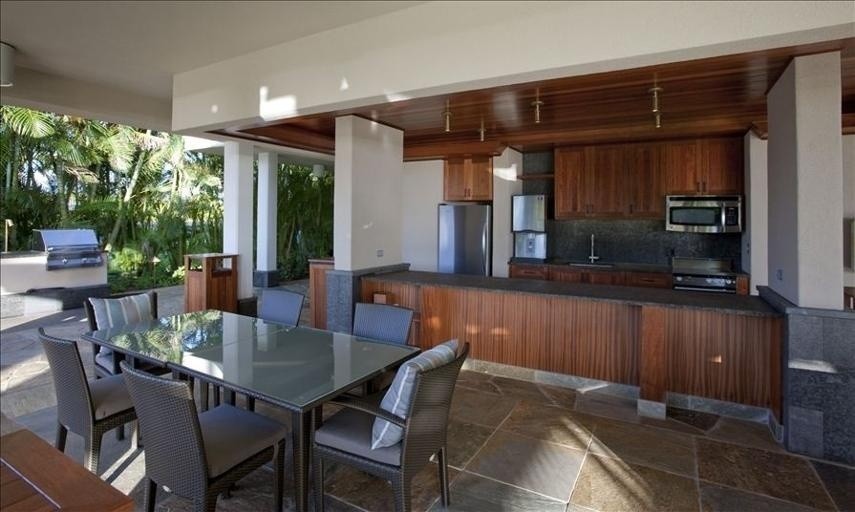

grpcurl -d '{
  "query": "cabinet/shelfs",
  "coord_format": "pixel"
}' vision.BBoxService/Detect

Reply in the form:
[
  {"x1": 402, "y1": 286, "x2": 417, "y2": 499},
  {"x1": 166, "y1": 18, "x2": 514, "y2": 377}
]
[
  {"x1": 510, "y1": 264, "x2": 674, "y2": 288},
  {"x1": 666, "y1": 137, "x2": 744, "y2": 196},
  {"x1": 554, "y1": 144, "x2": 626, "y2": 218},
  {"x1": 626, "y1": 142, "x2": 665, "y2": 217},
  {"x1": 444, "y1": 156, "x2": 492, "y2": 201}
]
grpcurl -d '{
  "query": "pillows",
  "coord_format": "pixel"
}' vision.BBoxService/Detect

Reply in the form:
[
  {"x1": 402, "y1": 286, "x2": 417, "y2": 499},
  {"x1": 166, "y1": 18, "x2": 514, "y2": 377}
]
[
  {"x1": 87, "y1": 288, "x2": 156, "y2": 356},
  {"x1": 372, "y1": 338, "x2": 459, "y2": 450}
]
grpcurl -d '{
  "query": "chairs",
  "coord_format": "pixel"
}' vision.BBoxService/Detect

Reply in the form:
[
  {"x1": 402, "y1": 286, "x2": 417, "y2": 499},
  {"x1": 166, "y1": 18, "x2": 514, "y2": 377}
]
[
  {"x1": 351, "y1": 302, "x2": 415, "y2": 397},
  {"x1": 119, "y1": 360, "x2": 286, "y2": 511},
  {"x1": 212, "y1": 288, "x2": 305, "y2": 411},
  {"x1": 37, "y1": 327, "x2": 138, "y2": 474},
  {"x1": 84, "y1": 290, "x2": 172, "y2": 441},
  {"x1": 311, "y1": 342, "x2": 469, "y2": 512}
]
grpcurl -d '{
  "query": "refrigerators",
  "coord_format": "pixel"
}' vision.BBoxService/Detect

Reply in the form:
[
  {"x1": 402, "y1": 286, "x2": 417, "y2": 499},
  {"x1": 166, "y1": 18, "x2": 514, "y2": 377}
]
[{"x1": 437, "y1": 204, "x2": 491, "y2": 277}]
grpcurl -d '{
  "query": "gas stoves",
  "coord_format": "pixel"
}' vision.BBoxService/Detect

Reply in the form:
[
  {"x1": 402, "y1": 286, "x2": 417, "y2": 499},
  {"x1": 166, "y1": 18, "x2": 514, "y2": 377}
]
[{"x1": 672, "y1": 267, "x2": 737, "y2": 289}]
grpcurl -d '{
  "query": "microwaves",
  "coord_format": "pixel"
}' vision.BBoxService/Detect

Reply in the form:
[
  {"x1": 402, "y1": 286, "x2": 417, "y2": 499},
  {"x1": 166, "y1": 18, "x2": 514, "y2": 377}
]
[{"x1": 665, "y1": 195, "x2": 742, "y2": 233}]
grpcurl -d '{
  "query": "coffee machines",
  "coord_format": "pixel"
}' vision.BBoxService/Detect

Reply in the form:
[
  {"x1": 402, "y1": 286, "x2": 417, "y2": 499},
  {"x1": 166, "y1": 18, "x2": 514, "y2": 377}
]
[{"x1": 510, "y1": 195, "x2": 548, "y2": 263}]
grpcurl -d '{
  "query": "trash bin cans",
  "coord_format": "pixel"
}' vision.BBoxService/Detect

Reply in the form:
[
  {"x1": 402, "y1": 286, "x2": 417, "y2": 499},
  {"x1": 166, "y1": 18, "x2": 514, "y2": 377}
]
[{"x1": 183, "y1": 252, "x2": 239, "y2": 314}]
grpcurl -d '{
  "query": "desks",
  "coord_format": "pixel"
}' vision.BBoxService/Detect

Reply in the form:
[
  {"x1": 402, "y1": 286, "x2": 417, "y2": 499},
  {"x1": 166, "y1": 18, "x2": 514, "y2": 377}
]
[
  {"x1": 0, "y1": 413, "x2": 136, "y2": 511},
  {"x1": 80, "y1": 308, "x2": 422, "y2": 510}
]
